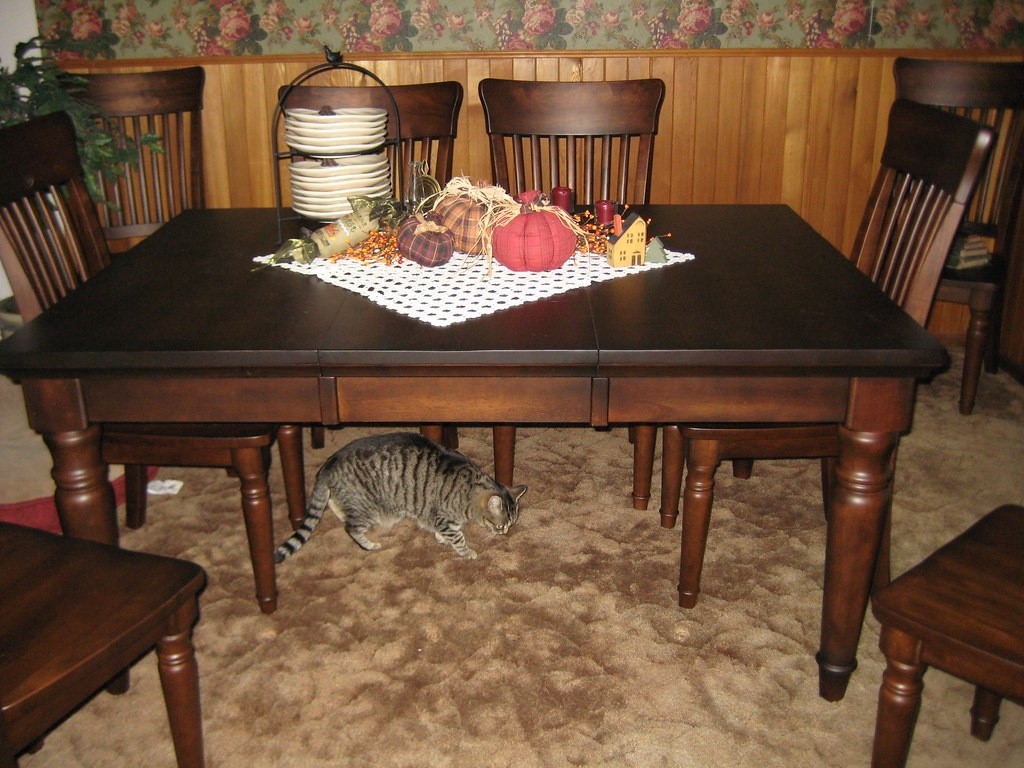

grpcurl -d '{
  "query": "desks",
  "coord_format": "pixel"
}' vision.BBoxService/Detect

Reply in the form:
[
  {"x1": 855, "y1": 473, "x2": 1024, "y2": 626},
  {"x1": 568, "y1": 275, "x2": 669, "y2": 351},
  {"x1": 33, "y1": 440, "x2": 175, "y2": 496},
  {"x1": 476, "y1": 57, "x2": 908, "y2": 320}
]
[{"x1": 0, "y1": 203, "x2": 953, "y2": 702}]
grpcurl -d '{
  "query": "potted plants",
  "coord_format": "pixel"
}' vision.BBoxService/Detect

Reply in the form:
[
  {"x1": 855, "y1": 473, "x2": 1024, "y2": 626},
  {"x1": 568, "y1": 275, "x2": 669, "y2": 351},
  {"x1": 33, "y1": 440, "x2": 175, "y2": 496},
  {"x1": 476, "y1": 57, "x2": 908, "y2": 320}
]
[{"x1": 1, "y1": 37, "x2": 165, "y2": 335}]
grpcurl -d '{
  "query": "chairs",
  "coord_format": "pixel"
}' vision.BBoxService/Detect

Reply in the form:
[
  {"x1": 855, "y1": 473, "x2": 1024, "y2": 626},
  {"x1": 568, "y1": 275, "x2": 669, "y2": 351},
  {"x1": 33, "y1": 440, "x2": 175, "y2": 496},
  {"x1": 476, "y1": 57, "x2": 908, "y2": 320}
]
[
  {"x1": 0, "y1": 112, "x2": 306, "y2": 614},
  {"x1": 478, "y1": 78, "x2": 663, "y2": 510},
  {"x1": 0, "y1": 518, "x2": 206, "y2": 768},
  {"x1": 893, "y1": 57, "x2": 1024, "y2": 415},
  {"x1": 871, "y1": 504, "x2": 1024, "y2": 768},
  {"x1": 57, "y1": 66, "x2": 208, "y2": 241},
  {"x1": 658, "y1": 98, "x2": 995, "y2": 608},
  {"x1": 274, "y1": 81, "x2": 464, "y2": 444}
]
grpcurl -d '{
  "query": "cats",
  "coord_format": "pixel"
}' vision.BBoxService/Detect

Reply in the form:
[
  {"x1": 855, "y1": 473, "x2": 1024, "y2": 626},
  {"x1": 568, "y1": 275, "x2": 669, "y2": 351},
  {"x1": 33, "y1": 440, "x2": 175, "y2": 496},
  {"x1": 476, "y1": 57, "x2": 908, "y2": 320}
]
[{"x1": 274, "y1": 431, "x2": 528, "y2": 565}]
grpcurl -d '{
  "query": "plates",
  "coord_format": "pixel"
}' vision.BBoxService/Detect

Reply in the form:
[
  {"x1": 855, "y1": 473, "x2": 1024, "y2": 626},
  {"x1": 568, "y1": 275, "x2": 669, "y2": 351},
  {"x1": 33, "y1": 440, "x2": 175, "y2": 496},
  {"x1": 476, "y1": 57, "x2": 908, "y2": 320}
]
[{"x1": 284, "y1": 107, "x2": 392, "y2": 225}]
